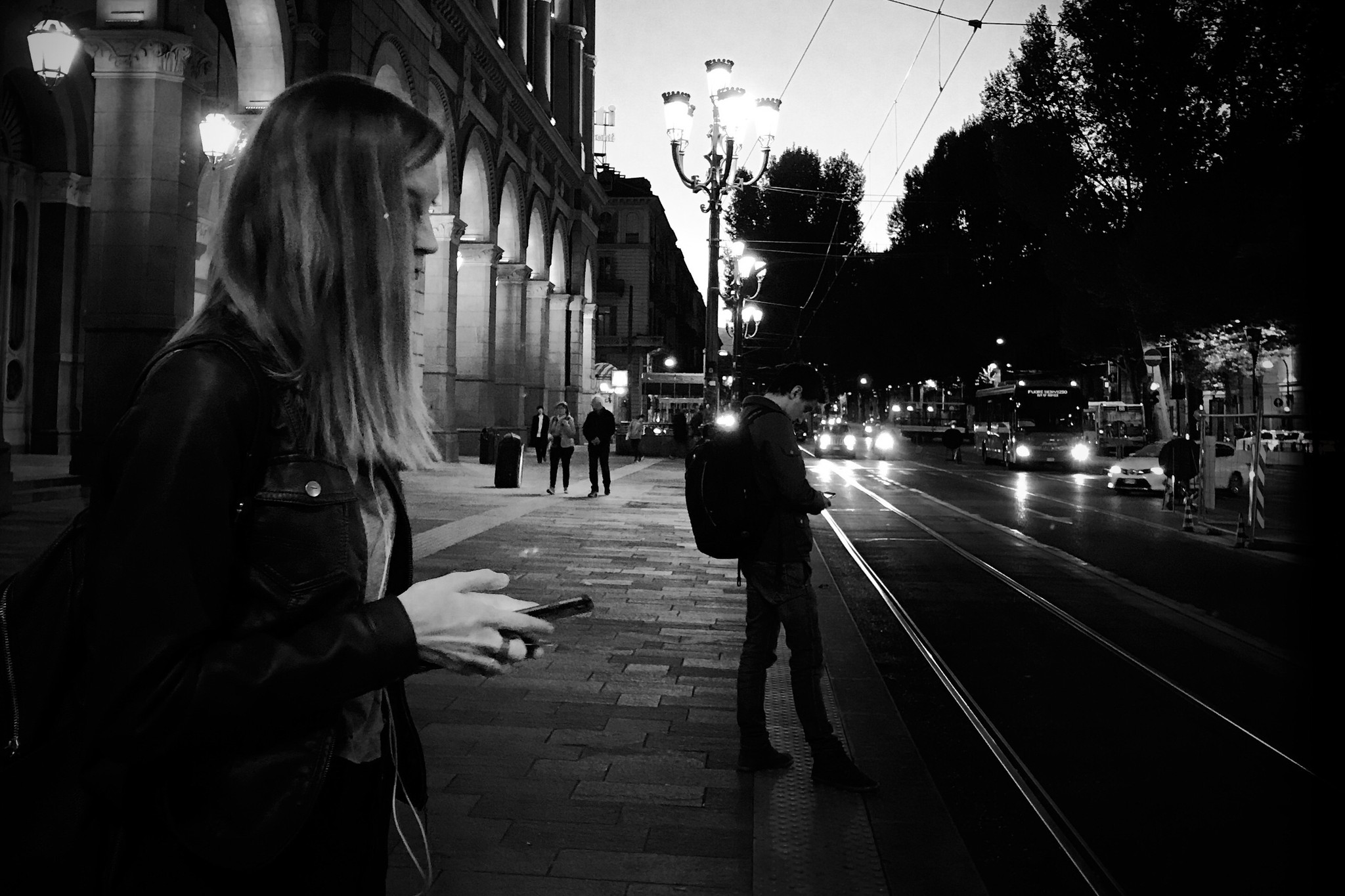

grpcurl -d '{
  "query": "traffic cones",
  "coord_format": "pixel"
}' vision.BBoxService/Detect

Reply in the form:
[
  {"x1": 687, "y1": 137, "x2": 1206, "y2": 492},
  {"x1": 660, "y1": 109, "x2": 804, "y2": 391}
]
[
  {"x1": 1179, "y1": 500, "x2": 1195, "y2": 532},
  {"x1": 1234, "y1": 512, "x2": 1245, "y2": 548}
]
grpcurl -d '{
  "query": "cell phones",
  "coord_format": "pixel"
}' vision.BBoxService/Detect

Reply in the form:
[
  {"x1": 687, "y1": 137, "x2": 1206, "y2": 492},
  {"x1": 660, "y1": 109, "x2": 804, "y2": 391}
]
[
  {"x1": 512, "y1": 593, "x2": 595, "y2": 622},
  {"x1": 823, "y1": 492, "x2": 837, "y2": 500}
]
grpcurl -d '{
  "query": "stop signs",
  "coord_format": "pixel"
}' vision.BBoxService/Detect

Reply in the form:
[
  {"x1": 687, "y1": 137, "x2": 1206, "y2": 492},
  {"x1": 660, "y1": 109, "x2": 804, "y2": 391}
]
[{"x1": 1144, "y1": 349, "x2": 1162, "y2": 367}]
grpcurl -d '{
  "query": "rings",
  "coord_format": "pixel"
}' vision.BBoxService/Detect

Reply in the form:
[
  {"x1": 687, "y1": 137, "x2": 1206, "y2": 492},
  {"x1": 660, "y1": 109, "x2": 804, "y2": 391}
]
[{"x1": 496, "y1": 634, "x2": 513, "y2": 662}]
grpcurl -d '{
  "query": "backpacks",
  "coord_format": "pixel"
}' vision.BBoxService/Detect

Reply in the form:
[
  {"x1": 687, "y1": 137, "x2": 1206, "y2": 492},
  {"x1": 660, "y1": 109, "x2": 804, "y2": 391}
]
[
  {"x1": 684, "y1": 406, "x2": 788, "y2": 559},
  {"x1": 0, "y1": 334, "x2": 264, "y2": 896}
]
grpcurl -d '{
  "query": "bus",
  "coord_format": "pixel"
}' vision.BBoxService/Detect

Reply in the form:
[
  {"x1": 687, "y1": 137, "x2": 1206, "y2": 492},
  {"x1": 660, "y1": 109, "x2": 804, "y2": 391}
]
[
  {"x1": 1081, "y1": 400, "x2": 1147, "y2": 456},
  {"x1": 974, "y1": 380, "x2": 1088, "y2": 471},
  {"x1": 887, "y1": 398, "x2": 969, "y2": 440}
]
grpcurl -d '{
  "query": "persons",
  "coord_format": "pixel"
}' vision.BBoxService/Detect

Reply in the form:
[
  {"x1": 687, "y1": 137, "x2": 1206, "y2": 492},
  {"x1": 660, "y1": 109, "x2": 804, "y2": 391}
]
[
  {"x1": 546, "y1": 401, "x2": 575, "y2": 495},
  {"x1": 690, "y1": 403, "x2": 703, "y2": 442},
  {"x1": 625, "y1": 414, "x2": 646, "y2": 462},
  {"x1": 669, "y1": 408, "x2": 689, "y2": 460},
  {"x1": 582, "y1": 397, "x2": 616, "y2": 498},
  {"x1": 734, "y1": 365, "x2": 880, "y2": 791},
  {"x1": 530, "y1": 405, "x2": 550, "y2": 463},
  {"x1": 124, "y1": 70, "x2": 559, "y2": 896}
]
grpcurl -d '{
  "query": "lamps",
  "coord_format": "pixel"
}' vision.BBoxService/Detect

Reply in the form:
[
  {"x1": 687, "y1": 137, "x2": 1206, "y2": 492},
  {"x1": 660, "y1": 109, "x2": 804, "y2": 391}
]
[
  {"x1": 197, "y1": 21, "x2": 237, "y2": 172},
  {"x1": 23, "y1": 0, "x2": 85, "y2": 92}
]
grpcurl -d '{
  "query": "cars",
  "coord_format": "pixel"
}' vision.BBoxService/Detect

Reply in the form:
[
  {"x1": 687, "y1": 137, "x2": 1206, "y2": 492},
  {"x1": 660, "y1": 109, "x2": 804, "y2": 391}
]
[
  {"x1": 1107, "y1": 438, "x2": 1253, "y2": 498},
  {"x1": 814, "y1": 423, "x2": 856, "y2": 458}
]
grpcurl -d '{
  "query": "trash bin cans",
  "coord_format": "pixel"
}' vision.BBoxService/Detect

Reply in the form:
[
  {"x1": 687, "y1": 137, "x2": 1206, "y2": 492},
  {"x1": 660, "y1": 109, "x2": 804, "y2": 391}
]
[
  {"x1": 479, "y1": 427, "x2": 495, "y2": 465},
  {"x1": 494, "y1": 432, "x2": 525, "y2": 488}
]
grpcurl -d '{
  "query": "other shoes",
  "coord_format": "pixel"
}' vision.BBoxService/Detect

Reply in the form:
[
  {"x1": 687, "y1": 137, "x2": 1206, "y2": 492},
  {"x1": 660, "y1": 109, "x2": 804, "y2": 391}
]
[
  {"x1": 547, "y1": 487, "x2": 554, "y2": 494},
  {"x1": 736, "y1": 739, "x2": 795, "y2": 772},
  {"x1": 604, "y1": 484, "x2": 610, "y2": 496},
  {"x1": 811, "y1": 737, "x2": 881, "y2": 792},
  {"x1": 588, "y1": 490, "x2": 597, "y2": 498},
  {"x1": 563, "y1": 487, "x2": 568, "y2": 494}
]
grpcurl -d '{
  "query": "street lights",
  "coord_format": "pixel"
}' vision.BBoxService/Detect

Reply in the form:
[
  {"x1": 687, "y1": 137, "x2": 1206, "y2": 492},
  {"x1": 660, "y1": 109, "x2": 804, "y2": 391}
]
[
  {"x1": 660, "y1": 57, "x2": 781, "y2": 440},
  {"x1": 1261, "y1": 356, "x2": 1290, "y2": 414},
  {"x1": 727, "y1": 239, "x2": 767, "y2": 410}
]
